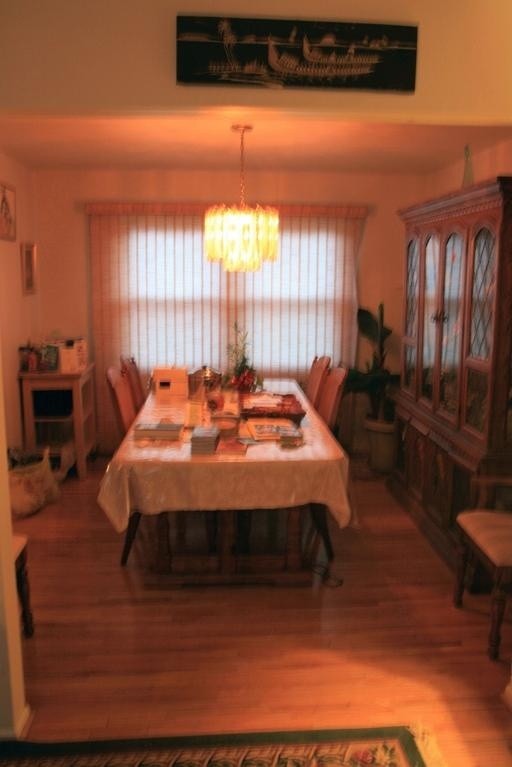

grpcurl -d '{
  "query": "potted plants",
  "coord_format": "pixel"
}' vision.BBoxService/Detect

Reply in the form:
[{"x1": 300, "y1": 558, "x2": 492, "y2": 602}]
[{"x1": 342, "y1": 301, "x2": 403, "y2": 477}]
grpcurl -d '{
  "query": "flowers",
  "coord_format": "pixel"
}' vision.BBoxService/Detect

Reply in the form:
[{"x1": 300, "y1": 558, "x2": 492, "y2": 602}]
[{"x1": 225, "y1": 325, "x2": 257, "y2": 390}]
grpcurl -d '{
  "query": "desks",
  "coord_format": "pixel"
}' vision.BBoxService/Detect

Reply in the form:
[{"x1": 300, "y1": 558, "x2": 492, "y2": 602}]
[
  {"x1": 21, "y1": 361, "x2": 98, "y2": 481},
  {"x1": 107, "y1": 376, "x2": 348, "y2": 568}
]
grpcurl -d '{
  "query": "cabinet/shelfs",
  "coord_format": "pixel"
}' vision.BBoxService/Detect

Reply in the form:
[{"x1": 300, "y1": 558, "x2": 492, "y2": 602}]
[{"x1": 389, "y1": 172, "x2": 511, "y2": 567}]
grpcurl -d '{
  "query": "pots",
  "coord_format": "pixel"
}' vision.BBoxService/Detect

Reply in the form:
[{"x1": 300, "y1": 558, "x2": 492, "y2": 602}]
[{"x1": 189, "y1": 365, "x2": 222, "y2": 400}]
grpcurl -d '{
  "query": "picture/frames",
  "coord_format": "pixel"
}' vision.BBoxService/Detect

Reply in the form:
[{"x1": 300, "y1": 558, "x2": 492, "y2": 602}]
[{"x1": 0, "y1": 182, "x2": 42, "y2": 298}]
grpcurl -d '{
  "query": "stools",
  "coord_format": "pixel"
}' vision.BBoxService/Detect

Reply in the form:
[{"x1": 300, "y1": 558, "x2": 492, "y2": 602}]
[{"x1": 450, "y1": 463, "x2": 512, "y2": 657}]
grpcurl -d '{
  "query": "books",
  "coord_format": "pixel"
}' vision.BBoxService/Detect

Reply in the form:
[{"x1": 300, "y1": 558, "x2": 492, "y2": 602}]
[{"x1": 132, "y1": 416, "x2": 304, "y2": 455}]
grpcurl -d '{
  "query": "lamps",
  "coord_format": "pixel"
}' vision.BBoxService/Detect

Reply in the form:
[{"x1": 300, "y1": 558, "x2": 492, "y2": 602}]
[{"x1": 201, "y1": 120, "x2": 283, "y2": 278}]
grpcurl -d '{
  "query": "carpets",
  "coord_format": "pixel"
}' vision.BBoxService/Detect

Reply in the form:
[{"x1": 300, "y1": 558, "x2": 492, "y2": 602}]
[{"x1": 0, "y1": 723, "x2": 428, "y2": 767}]
